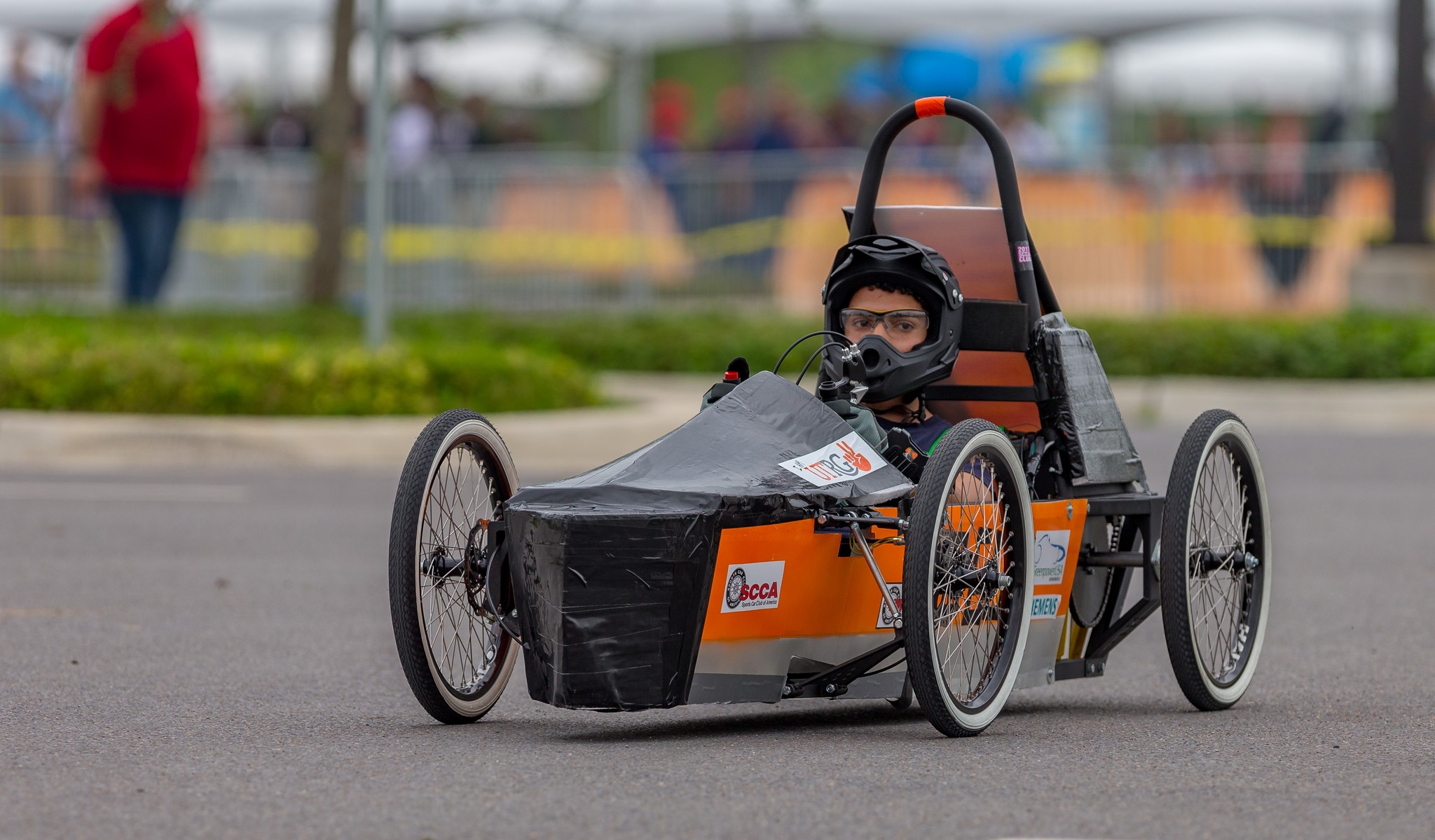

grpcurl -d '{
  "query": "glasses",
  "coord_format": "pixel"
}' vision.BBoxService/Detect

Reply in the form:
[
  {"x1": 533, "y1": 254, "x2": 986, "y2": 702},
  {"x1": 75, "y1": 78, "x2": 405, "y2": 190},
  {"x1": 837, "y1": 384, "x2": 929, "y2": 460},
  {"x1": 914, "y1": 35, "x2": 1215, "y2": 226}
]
[{"x1": 835, "y1": 308, "x2": 931, "y2": 339}]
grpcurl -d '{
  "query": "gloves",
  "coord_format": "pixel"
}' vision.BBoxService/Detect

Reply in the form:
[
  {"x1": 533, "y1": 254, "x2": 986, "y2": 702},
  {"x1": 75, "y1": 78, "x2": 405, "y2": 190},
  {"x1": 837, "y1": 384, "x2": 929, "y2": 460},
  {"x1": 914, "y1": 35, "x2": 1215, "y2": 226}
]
[{"x1": 702, "y1": 358, "x2": 750, "y2": 416}]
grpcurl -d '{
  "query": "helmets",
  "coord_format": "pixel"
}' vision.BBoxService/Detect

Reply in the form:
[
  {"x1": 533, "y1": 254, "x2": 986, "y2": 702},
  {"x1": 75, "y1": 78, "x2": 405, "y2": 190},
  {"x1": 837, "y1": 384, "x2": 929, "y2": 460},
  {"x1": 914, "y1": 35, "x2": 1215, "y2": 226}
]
[{"x1": 825, "y1": 233, "x2": 965, "y2": 401}]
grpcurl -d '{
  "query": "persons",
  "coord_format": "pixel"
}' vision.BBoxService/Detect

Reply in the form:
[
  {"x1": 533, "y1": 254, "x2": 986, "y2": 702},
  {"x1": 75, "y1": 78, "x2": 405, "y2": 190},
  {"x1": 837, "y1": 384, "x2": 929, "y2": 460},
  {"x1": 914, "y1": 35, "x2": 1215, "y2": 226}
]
[
  {"x1": 6, "y1": 32, "x2": 1434, "y2": 315},
  {"x1": 803, "y1": 233, "x2": 998, "y2": 513},
  {"x1": 66, "y1": 2, "x2": 210, "y2": 307}
]
